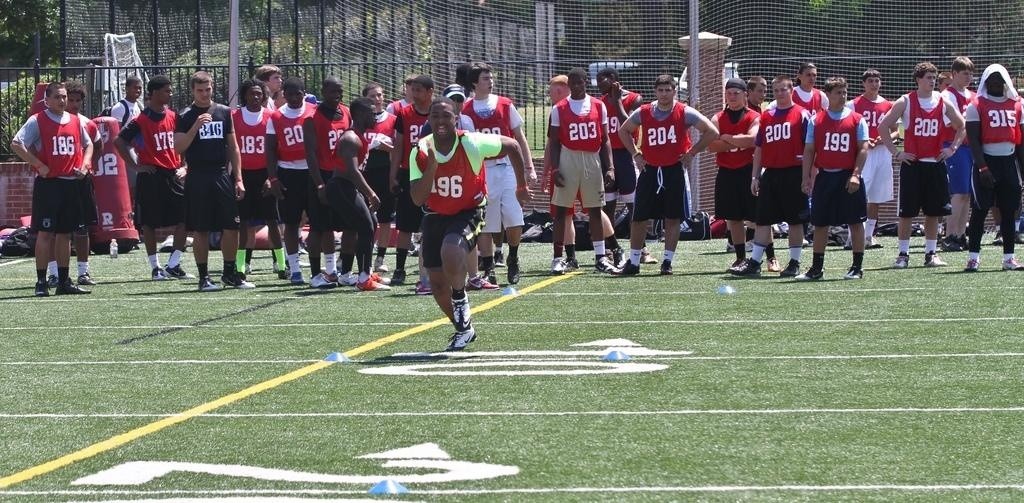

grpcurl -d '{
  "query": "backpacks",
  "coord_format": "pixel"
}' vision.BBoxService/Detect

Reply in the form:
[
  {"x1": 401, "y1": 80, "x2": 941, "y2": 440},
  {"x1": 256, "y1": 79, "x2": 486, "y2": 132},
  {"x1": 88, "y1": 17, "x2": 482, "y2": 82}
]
[{"x1": 0, "y1": 225, "x2": 31, "y2": 255}]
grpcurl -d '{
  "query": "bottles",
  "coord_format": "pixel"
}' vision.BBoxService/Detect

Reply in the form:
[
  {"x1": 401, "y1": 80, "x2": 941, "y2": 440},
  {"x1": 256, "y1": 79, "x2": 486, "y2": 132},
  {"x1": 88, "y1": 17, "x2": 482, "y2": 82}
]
[{"x1": 108, "y1": 237, "x2": 117, "y2": 259}]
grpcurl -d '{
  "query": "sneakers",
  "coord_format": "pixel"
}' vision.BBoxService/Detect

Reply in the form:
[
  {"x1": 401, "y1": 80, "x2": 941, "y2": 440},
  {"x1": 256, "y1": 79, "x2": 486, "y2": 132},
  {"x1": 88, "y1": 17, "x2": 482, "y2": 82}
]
[
  {"x1": 144, "y1": 250, "x2": 432, "y2": 295},
  {"x1": 451, "y1": 291, "x2": 471, "y2": 332},
  {"x1": 33, "y1": 271, "x2": 104, "y2": 295},
  {"x1": 550, "y1": 246, "x2": 688, "y2": 276},
  {"x1": 443, "y1": 327, "x2": 484, "y2": 352},
  {"x1": 466, "y1": 257, "x2": 520, "y2": 290},
  {"x1": 722, "y1": 229, "x2": 1023, "y2": 282}
]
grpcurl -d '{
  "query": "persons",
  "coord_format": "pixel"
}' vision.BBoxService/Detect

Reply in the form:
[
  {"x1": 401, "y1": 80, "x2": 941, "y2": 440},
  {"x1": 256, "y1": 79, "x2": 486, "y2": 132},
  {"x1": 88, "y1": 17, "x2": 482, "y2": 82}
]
[
  {"x1": 539, "y1": 66, "x2": 719, "y2": 276},
  {"x1": 413, "y1": 100, "x2": 536, "y2": 351},
  {"x1": 11, "y1": 82, "x2": 104, "y2": 297},
  {"x1": 111, "y1": 63, "x2": 536, "y2": 291},
  {"x1": 709, "y1": 55, "x2": 1024, "y2": 278}
]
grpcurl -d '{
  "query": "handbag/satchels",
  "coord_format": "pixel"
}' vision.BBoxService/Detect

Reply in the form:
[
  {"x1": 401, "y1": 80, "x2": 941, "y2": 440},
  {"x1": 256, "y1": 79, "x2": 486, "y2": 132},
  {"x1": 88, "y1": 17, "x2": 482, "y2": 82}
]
[{"x1": 522, "y1": 209, "x2": 726, "y2": 244}]
